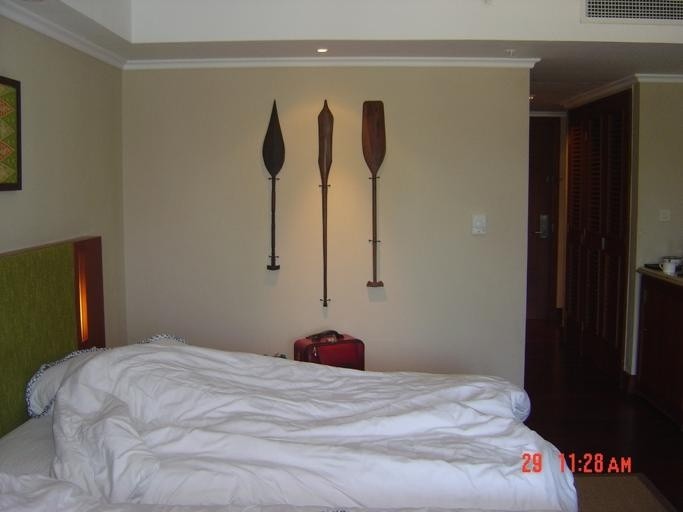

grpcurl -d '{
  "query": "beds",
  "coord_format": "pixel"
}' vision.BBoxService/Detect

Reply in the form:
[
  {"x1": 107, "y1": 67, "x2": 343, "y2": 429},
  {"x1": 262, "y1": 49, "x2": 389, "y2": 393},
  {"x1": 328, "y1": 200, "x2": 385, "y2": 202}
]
[{"x1": 0, "y1": 237, "x2": 578, "y2": 512}]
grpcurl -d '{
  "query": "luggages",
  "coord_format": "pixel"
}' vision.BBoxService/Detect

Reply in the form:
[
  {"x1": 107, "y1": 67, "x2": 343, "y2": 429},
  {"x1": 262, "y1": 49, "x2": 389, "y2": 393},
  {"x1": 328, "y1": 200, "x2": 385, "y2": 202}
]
[{"x1": 294, "y1": 329, "x2": 364, "y2": 371}]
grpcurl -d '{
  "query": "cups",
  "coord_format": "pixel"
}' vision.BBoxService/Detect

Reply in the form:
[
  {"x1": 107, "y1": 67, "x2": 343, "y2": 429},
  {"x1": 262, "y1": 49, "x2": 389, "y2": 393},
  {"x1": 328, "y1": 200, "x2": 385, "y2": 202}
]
[{"x1": 659, "y1": 260, "x2": 675, "y2": 276}]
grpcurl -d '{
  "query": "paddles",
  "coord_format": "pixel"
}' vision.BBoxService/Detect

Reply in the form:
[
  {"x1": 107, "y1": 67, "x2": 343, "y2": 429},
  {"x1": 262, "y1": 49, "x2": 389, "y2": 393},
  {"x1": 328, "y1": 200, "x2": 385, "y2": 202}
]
[
  {"x1": 318, "y1": 100, "x2": 334, "y2": 307},
  {"x1": 262, "y1": 100, "x2": 285, "y2": 271},
  {"x1": 361, "y1": 101, "x2": 386, "y2": 288}
]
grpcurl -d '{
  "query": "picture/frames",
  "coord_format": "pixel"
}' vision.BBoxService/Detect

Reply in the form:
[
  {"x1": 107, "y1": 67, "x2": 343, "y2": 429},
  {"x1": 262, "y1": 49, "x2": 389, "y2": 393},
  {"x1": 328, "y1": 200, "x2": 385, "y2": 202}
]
[{"x1": 0, "y1": 75, "x2": 23, "y2": 192}]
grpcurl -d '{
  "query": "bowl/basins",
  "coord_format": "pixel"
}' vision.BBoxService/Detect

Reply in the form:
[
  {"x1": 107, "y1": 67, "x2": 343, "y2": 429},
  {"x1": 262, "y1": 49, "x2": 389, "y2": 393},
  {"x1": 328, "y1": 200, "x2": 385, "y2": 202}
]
[{"x1": 664, "y1": 256, "x2": 683, "y2": 266}]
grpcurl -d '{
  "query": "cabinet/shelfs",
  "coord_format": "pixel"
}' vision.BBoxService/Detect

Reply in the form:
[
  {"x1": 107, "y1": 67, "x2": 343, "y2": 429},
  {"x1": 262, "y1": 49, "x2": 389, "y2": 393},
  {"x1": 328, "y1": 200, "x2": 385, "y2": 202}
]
[
  {"x1": 567, "y1": 84, "x2": 634, "y2": 378},
  {"x1": 630, "y1": 263, "x2": 683, "y2": 512}
]
